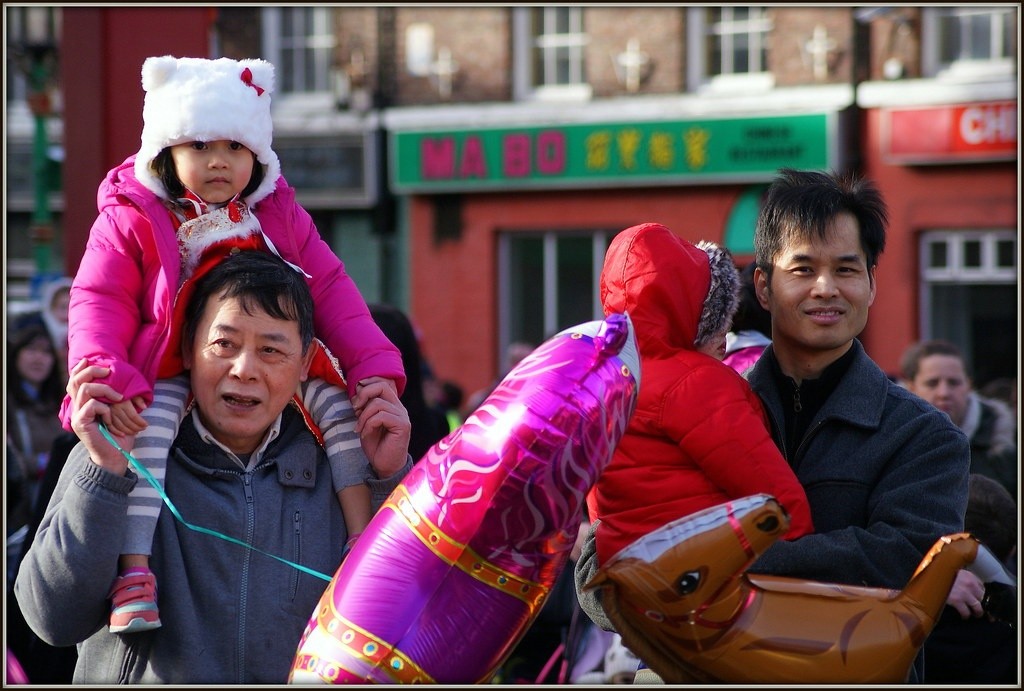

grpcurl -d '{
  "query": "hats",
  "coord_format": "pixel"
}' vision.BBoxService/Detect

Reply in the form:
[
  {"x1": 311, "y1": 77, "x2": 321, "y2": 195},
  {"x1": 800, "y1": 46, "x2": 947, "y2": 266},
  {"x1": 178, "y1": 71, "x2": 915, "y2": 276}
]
[
  {"x1": 43, "y1": 279, "x2": 76, "y2": 342},
  {"x1": 133, "y1": 55, "x2": 281, "y2": 208}
]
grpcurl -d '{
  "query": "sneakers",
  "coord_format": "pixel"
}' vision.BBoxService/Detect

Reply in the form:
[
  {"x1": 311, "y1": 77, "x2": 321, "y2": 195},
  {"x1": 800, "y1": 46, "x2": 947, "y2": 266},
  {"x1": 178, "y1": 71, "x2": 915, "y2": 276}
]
[
  {"x1": 106, "y1": 571, "x2": 162, "y2": 633},
  {"x1": 632, "y1": 660, "x2": 665, "y2": 685},
  {"x1": 341, "y1": 533, "x2": 362, "y2": 563}
]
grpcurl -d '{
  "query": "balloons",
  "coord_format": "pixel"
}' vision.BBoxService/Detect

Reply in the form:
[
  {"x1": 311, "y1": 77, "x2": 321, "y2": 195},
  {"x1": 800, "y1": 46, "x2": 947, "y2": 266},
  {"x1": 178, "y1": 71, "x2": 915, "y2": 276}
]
[
  {"x1": 581, "y1": 495, "x2": 980, "y2": 686},
  {"x1": 285, "y1": 313, "x2": 640, "y2": 684}
]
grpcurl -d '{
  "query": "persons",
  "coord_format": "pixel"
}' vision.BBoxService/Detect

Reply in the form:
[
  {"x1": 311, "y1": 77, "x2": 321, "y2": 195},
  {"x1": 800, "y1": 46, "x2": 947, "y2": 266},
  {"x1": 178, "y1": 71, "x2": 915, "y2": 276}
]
[
  {"x1": 58, "y1": 56, "x2": 408, "y2": 635},
  {"x1": 7, "y1": 277, "x2": 74, "y2": 592},
  {"x1": 893, "y1": 338, "x2": 1018, "y2": 687},
  {"x1": 573, "y1": 164, "x2": 973, "y2": 626},
  {"x1": 15, "y1": 248, "x2": 417, "y2": 685},
  {"x1": 367, "y1": 300, "x2": 536, "y2": 464},
  {"x1": 586, "y1": 223, "x2": 814, "y2": 569}
]
